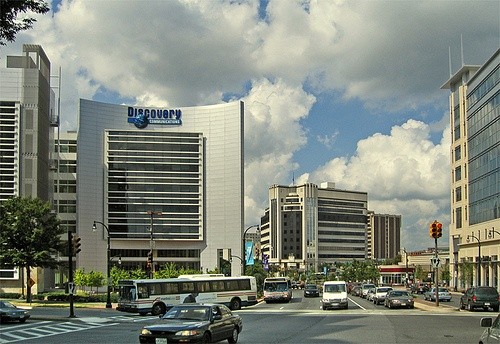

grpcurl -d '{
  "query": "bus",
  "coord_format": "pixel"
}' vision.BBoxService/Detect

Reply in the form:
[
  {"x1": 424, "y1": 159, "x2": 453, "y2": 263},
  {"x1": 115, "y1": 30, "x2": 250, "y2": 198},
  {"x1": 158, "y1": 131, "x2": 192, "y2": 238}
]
[
  {"x1": 263, "y1": 277, "x2": 292, "y2": 303},
  {"x1": 116, "y1": 274, "x2": 257, "y2": 314}
]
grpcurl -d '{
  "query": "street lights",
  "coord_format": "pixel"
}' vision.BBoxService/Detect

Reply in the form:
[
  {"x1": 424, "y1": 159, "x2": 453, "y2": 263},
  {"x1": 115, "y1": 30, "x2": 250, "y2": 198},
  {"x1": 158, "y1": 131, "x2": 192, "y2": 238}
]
[
  {"x1": 229, "y1": 224, "x2": 261, "y2": 276},
  {"x1": 148, "y1": 210, "x2": 162, "y2": 278},
  {"x1": 465, "y1": 234, "x2": 492, "y2": 286},
  {"x1": 91, "y1": 220, "x2": 122, "y2": 308}
]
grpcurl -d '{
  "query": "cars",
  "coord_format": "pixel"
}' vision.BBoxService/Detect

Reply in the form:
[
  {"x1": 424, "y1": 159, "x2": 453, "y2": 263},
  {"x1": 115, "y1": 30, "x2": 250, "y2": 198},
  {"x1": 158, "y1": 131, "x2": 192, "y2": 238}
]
[
  {"x1": 140, "y1": 304, "x2": 243, "y2": 344},
  {"x1": 304, "y1": 284, "x2": 319, "y2": 297},
  {"x1": 347, "y1": 282, "x2": 394, "y2": 305},
  {"x1": 384, "y1": 290, "x2": 414, "y2": 309},
  {"x1": 410, "y1": 283, "x2": 429, "y2": 294},
  {"x1": 0, "y1": 301, "x2": 30, "y2": 323},
  {"x1": 424, "y1": 287, "x2": 452, "y2": 302},
  {"x1": 291, "y1": 281, "x2": 304, "y2": 290}
]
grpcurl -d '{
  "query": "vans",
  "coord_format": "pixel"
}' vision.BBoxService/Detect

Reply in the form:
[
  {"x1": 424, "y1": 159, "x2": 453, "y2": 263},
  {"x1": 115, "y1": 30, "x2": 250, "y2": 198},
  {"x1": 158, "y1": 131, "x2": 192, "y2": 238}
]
[
  {"x1": 323, "y1": 281, "x2": 348, "y2": 309},
  {"x1": 478, "y1": 314, "x2": 500, "y2": 344}
]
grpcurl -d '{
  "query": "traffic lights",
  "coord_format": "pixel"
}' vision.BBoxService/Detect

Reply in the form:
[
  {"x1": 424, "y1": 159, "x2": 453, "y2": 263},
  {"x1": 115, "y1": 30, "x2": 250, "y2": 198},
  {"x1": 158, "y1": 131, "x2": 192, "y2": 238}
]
[
  {"x1": 72, "y1": 235, "x2": 81, "y2": 257},
  {"x1": 430, "y1": 222, "x2": 442, "y2": 238},
  {"x1": 148, "y1": 253, "x2": 152, "y2": 262}
]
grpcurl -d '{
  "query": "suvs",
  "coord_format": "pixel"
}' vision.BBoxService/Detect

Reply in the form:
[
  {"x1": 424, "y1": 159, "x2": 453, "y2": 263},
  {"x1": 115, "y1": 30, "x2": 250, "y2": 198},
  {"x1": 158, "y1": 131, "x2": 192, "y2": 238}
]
[{"x1": 460, "y1": 286, "x2": 500, "y2": 312}]
